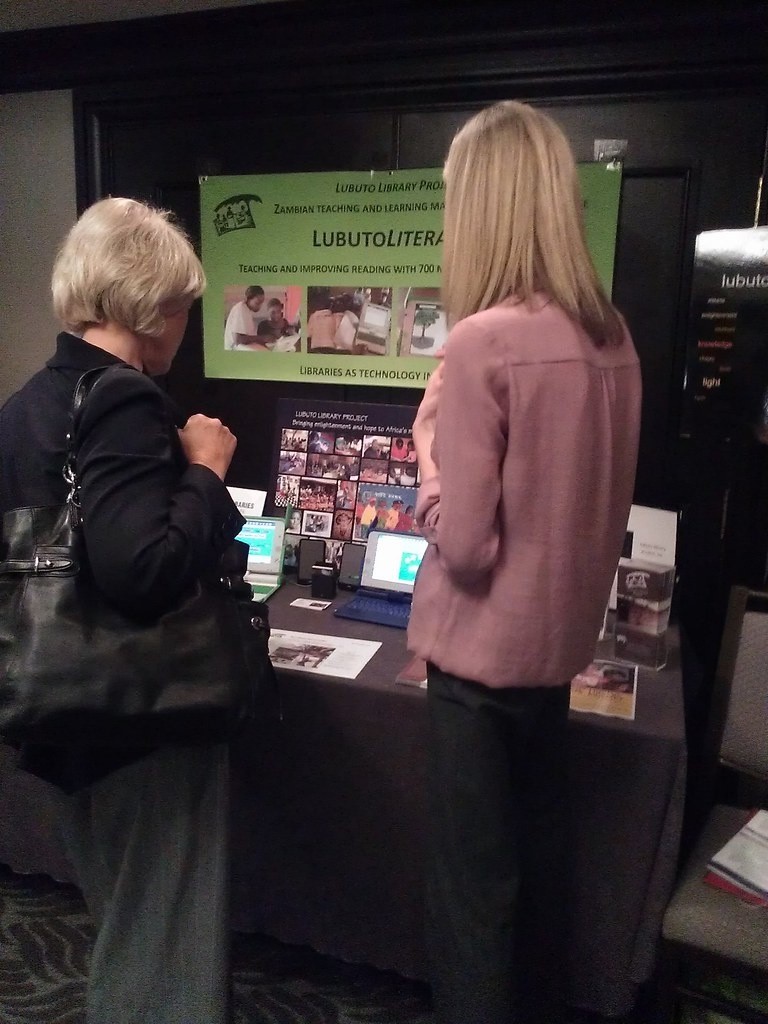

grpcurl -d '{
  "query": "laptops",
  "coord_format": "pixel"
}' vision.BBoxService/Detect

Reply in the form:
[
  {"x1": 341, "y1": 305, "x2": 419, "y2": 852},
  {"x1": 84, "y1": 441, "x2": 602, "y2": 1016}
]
[
  {"x1": 235, "y1": 504, "x2": 294, "y2": 604},
  {"x1": 334, "y1": 527, "x2": 429, "y2": 629}
]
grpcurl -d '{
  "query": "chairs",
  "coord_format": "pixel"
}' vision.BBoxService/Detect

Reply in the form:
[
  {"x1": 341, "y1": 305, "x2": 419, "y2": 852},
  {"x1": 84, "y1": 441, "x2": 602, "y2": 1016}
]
[{"x1": 651, "y1": 585, "x2": 768, "y2": 1024}]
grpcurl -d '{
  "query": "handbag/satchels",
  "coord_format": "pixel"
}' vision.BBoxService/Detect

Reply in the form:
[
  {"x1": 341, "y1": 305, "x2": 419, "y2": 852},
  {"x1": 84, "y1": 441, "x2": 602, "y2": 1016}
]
[{"x1": 0, "y1": 363, "x2": 284, "y2": 745}]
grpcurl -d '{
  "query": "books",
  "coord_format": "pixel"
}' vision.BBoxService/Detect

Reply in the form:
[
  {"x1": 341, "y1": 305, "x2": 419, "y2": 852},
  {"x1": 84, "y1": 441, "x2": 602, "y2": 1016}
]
[{"x1": 395, "y1": 653, "x2": 428, "y2": 689}]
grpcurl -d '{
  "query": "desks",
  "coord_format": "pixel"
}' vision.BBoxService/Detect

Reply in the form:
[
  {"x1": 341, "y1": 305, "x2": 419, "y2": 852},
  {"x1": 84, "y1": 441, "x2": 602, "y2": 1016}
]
[{"x1": 0, "y1": 581, "x2": 685, "y2": 1013}]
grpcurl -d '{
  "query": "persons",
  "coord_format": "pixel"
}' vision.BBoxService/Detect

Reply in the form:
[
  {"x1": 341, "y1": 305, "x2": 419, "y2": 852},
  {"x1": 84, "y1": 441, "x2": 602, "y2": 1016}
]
[
  {"x1": 348, "y1": 438, "x2": 362, "y2": 456},
  {"x1": 281, "y1": 429, "x2": 307, "y2": 451},
  {"x1": 337, "y1": 482, "x2": 357, "y2": 509},
  {"x1": 390, "y1": 440, "x2": 417, "y2": 462},
  {"x1": 285, "y1": 509, "x2": 302, "y2": 534},
  {"x1": 357, "y1": 494, "x2": 413, "y2": 539},
  {"x1": 411, "y1": 101, "x2": 642, "y2": 1024},
  {"x1": 280, "y1": 479, "x2": 297, "y2": 497},
  {"x1": 389, "y1": 465, "x2": 416, "y2": 485},
  {"x1": 256, "y1": 298, "x2": 290, "y2": 343},
  {"x1": 307, "y1": 456, "x2": 359, "y2": 480},
  {"x1": 307, "y1": 293, "x2": 360, "y2": 354},
  {"x1": 282, "y1": 456, "x2": 304, "y2": 472},
  {"x1": 309, "y1": 432, "x2": 333, "y2": 452},
  {"x1": 301, "y1": 483, "x2": 336, "y2": 509},
  {"x1": 362, "y1": 466, "x2": 386, "y2": 482},
  {"x1": 225, "y1": 285, "x2": 275, "y2": 351},
  {"x1": 364, "y1": 439, "x2": 390, "y2": 459},
  {"x1": 0, "y1": 197, "x2": 236, "y2": 1024},
  {"x1": 284, "y1": 511, "x2": 349, "y2": 566}
]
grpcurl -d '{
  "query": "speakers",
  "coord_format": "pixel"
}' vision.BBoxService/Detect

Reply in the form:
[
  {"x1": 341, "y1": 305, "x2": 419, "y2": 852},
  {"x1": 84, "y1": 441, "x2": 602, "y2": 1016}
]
[
  {"x1": 338, "y1": 541, "x2": 365, "y2": 591},
  {"x1": 297, "y1": 538, "x2": 327, "y2": 585}
]
